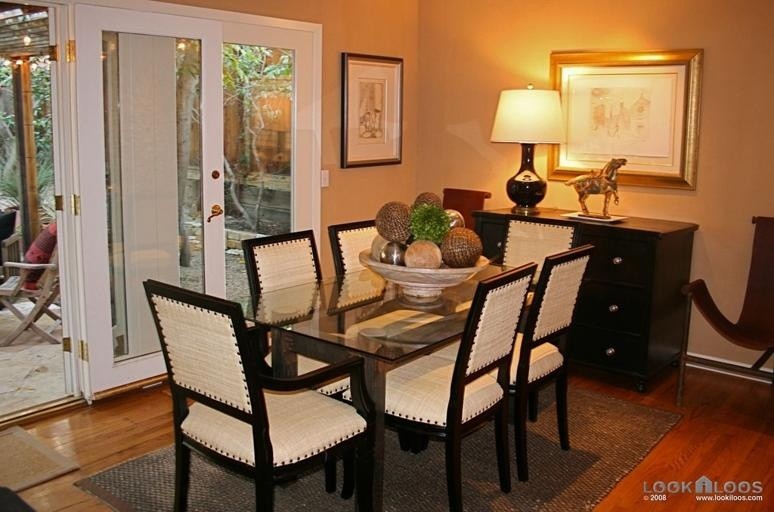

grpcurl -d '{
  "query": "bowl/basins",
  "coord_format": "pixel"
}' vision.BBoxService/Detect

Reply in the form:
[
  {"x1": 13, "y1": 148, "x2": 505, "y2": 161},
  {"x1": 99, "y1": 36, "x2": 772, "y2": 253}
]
[{"x1": 359, "y1": 249, "x2": 489, "y2": 299}]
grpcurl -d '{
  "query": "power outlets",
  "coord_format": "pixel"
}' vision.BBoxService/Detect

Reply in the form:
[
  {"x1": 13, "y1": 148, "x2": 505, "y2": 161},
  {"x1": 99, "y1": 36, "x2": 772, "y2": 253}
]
[{"x1": 321, "y1": 170, "x2": 331, "y2": 189}]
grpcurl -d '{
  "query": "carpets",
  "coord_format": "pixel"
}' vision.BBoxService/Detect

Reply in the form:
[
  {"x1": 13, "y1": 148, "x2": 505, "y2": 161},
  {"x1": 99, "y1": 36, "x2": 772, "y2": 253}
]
[
  {"x1": 73, "y1": 385, "x2": 682, "y2": 511},
  {"x1": 0, "y1": 425, "x2": 82, "y2": 493}
]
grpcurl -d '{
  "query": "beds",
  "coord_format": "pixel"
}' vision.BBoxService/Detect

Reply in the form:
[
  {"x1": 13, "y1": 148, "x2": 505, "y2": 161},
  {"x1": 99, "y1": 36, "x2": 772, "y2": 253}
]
[{"x1": 228, "y1": 264, "x2": 541, "y2": 511}]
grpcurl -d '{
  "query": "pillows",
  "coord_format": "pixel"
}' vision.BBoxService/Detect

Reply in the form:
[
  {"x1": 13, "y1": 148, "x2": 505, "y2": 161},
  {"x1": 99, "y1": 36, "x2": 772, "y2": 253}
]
[{"x1": 21, "y1": 223, "x2": 57, "y2": 290}]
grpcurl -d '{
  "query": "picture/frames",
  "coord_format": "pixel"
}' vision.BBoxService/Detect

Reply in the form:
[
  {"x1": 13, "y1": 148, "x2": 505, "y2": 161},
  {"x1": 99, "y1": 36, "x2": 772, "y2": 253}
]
[
  {"x1": 548, "y1": 48, "x2": 704, "y2": 196},
  {"x1": 340, "y1": 52, "x2": 404, "y2": 168},
  {"x1": 489, "y1": 84, "x2": 566, "y2": 215}
]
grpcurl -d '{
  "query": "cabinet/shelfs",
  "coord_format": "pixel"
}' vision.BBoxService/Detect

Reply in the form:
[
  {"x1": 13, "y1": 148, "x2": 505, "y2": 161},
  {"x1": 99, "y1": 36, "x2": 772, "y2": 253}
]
[{"x1": 468, "y1": 208, "x2": 699, "y2": 394}]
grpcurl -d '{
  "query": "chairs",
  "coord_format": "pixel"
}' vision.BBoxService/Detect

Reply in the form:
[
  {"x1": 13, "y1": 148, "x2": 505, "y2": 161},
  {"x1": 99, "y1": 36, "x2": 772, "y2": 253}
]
[
  {"x1": 442, "y1": 188, "x2": 492, "y2": 223},
  {"x1": 0, "y1": 218, "x2": 61, "y2": 346},
  {"x1": 342, "y1": 263, "x2": 539, "y2": 512},
  {"x1": 427, "y1": 243, "x2": 599, "y2": 482},
  {"x1": 143, "y1": 279, "x2": 377, "y2": 512},
  {"x1": 241, "y1": 230, "x2": 322, "y2": 295},
  {"x1": 328, "y1": 219, "x2": 379, "y2": 277},
  {"x1": 676, "y1": 215, "x2": 774, "y2": 409}
]
